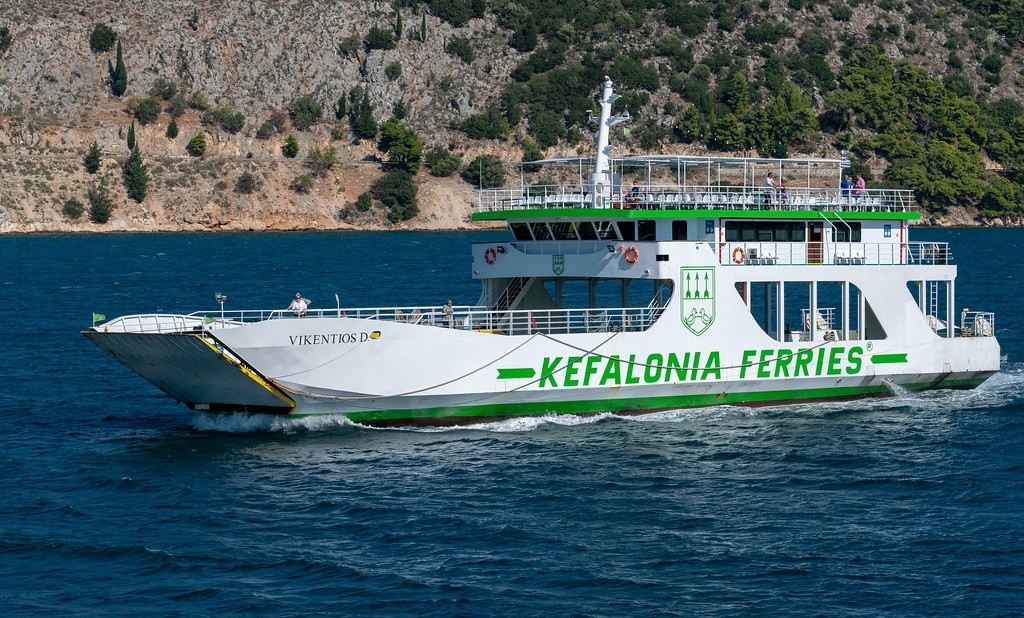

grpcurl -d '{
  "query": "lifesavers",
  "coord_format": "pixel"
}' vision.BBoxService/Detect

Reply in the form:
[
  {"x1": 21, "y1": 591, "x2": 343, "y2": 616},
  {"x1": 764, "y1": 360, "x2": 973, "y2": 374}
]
[
  {"x1": 928, "y1": 245, "x2": 938, "y2": 257},
  {"x1": 484, "y1": 248, "x2": 496, "y2": 264},
  {"x1": 625, "y1": 246, "x2": 639, "y2": 264},
  {"x1": 732, "y1": 247, "x2": 745, "y2": 264}
]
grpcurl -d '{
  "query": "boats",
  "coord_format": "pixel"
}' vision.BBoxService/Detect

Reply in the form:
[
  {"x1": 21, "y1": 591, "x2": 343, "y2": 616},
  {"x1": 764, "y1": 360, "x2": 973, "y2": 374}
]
[{"x1": 78, "y1": 70, "x2": 1004, "y2": 421}]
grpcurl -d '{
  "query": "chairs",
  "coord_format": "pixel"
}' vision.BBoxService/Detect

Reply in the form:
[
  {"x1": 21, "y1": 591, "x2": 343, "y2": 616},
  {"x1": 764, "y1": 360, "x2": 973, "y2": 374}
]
[
  {"x1": 834, "y1": 257, "x2": 867, "y2": 264},
  {"x1": 743, "y1": 257, "x2": 779, "y2": 265},
  {"x1": 511, "y1": 193, "x2": 893, "y2": 212}
]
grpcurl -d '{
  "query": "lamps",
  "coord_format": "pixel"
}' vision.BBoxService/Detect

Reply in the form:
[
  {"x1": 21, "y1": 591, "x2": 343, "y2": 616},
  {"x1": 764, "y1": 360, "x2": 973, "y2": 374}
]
[
  {"x1": 607, "y1": 244, "x2": 615, "y2": 252},
  {"x1": 214, "y1": 292, "x2": 227, "y2": 303},
  {"x1": 497, "y1": 246, "x2": 506, "y2": 254}
]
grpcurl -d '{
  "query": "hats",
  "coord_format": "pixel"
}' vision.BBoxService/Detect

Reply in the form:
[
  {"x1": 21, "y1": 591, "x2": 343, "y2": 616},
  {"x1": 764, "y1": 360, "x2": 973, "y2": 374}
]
[{"x1": 781, "y1": 177, "x2": 786, "y2": 181}]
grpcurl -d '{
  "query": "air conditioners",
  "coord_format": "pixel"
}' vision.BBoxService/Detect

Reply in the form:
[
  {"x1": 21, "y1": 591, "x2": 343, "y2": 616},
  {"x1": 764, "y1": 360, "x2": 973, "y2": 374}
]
[{"x1": 590, "y1": 180, "x2": 611, "y2": 209}]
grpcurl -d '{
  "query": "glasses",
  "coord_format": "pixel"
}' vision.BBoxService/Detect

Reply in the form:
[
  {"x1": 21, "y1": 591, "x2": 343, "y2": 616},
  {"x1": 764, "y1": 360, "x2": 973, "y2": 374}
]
[{"x1": 296, "y1": 298, "x2": 300, "y2": 299}]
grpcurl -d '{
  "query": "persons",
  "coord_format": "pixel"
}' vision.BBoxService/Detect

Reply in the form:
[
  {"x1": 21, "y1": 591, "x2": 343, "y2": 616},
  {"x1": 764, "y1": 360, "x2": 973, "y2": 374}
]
[
  {"x1": 764, "y1": 172, "x2": 778, "y2": 209},
  {"x1": 626, "y1": 181, "x2": 639, "y2": 210},
  {"x1": 782, "y1": 176, "x2": 788, "y2": 203},
  {"x1": 286, "y1": 292, "x2": 310, "y2": 317},
  {"x1": 841, "y1": 171, "x2": 866, "y2": 211},
  {"x1": 442, "y1": 300, "x2": 454, "y2": 328}
]
